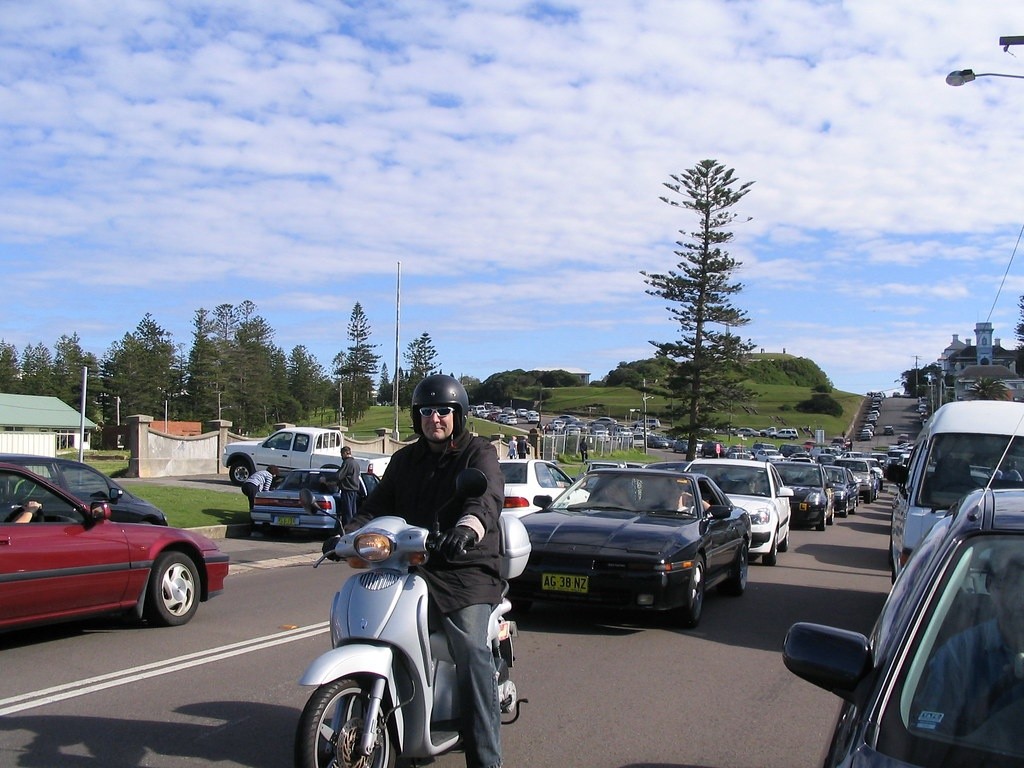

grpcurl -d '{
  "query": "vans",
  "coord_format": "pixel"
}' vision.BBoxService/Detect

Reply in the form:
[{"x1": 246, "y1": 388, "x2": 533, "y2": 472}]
[
  {"x1": 0, "y1": 451, "x2": 169, "y2": 528},
  {"x1": 880, "y1": 399, "x2": 1024, "y2": 585}
]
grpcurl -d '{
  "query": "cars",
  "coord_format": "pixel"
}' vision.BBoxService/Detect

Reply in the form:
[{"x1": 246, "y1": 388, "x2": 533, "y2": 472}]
[
  {"x1": 0, "y1": 460, "x2": 230, "y2": 635},
  {"x1": 681, "y1": 456, "x2": 795, "y2": 567},
  {"x1": 248, "y1": 467, "x2": 382, "y2": 540},
  {"x1": 781, "y1": 487, "x2": 1024, "y2": 768},
  {"x1": 498, "y1": 458, "x2": 590, "y2": 520},
  {"x1": 503, "y1": 467, "x2": 753, "y2": 629},
  {"x1": 467, "y1": 390, "x2": 1007, "y2": 532}
]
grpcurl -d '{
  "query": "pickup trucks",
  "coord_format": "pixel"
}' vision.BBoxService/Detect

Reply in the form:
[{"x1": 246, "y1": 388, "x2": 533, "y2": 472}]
[{"x1": 222, "y1": 426, "x2": 392, "y2": 487}]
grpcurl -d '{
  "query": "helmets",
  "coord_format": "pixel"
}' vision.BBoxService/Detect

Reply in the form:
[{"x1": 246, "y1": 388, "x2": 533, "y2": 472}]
[{"x1": 411, "y1": 374, "x2": 469, "y2": 434}]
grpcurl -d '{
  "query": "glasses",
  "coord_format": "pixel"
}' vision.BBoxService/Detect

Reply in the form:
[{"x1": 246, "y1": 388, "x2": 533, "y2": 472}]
[{"x1": 419, "y1": 407, "x2": 456, "y2": 417}]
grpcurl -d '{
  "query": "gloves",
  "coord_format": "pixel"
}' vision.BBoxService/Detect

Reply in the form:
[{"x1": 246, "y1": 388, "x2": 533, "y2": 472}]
[
  {"x1": 432, "y1": 525, "x2": 478, "y2": 556},
  {"x1": 322, "y1": 537, "x2": 342, "y2": 562}
]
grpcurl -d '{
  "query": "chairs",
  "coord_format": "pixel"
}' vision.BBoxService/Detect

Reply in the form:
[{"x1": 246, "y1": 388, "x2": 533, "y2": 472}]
[{"x1": 934, "y1": 456, "x2": 971, "y2": 491}]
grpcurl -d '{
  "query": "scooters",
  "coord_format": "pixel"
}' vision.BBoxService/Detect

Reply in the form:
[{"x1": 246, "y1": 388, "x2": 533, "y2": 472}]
[{"x1": 292, "y1": 465, "x2": 534, "y2": 768}]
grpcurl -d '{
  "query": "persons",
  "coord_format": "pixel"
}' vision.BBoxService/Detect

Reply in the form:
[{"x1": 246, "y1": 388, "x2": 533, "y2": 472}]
[
  {"x1": 14, "y1": 501, "x2": 43, "y2": 523},
  {"x1": 321, "y1": 375, "x2": 508, "y2": 768},
  {"x1": 537, "y1": 422, "x2": 549, "y2": 434},
  {"x1": 319, "y1": 445, "x2": 361, "y2": 527},
  {"x1": 506, "y1": 435, "x2": 518, "y2": 459},
  {"x1": 842, "y1": 431, "x2": 846, "y2": 438},
  {"x1": 580, "y1": 437, "x2": 588, "y2": 462},
  {"x1": 517, "y1": 436, "x2": 528, "y2": 459},
  {"x1": 608, "y1": 481, "x2": 710, "y2": 514},
  {"x1": 744, "y1": 475, "x2": 765, "y2": 495},
  {"x1": 832, "y1": 471, "x2": 842, "y2": 483},
  {"x1": 242, "y1": 465, "x2": 279, "y2": 538},
  {"x1": 910, "y1": 546, "x2": 1024, "y2": 735}
]
